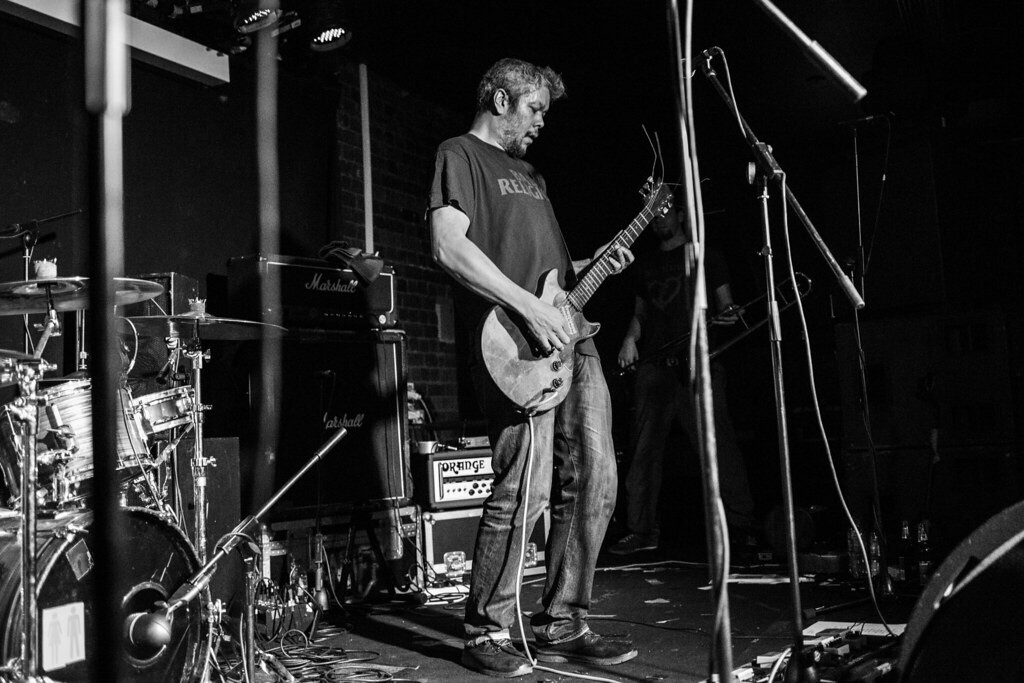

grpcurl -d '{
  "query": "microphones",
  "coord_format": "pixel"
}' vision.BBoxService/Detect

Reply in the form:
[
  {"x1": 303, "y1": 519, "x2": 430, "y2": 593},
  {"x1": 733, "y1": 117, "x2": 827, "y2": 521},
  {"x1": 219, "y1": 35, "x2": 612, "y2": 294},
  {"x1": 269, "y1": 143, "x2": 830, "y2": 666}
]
[
  {"x1": 123, "y1": 573, "x2": 212, "y2": 648},
  {"x1": 155, "y1": 361, "x2": 171, "y2": 385},
  {"x1": 681, "y1": 48, "x2": 718, "y2": 79},
  {"x1": 838, "y1": 115, "x2": 883, "y2": 124}
]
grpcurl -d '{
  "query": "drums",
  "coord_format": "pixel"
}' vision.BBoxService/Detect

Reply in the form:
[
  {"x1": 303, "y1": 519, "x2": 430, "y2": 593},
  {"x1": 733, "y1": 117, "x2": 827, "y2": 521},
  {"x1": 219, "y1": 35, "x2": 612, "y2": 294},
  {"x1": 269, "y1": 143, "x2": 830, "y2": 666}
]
[
  {"x1": 0, "y1": 377, "x2": 157, "y2": 514},
  {"x1": 128, "y1": 383, "x2": 200, "y2": 437},
  {"x1": 0, "y1": 506, "x2": 215, "y2": 683}
]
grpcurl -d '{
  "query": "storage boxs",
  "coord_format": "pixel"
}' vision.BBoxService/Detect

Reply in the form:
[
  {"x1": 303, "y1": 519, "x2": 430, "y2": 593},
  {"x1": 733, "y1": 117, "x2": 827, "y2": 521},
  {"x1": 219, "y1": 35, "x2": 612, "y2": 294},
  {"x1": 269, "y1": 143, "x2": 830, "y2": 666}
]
[{"x1": 262, "y1": 446, "x2": 550, "y2": 611}]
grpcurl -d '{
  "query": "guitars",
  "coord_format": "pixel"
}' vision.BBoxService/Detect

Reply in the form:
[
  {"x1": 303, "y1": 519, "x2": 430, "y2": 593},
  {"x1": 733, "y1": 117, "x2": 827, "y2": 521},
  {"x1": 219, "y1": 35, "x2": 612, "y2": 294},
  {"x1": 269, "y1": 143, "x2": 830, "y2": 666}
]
[{"x1": 478, "y1": 176, "x2": 676, "y2": 414}]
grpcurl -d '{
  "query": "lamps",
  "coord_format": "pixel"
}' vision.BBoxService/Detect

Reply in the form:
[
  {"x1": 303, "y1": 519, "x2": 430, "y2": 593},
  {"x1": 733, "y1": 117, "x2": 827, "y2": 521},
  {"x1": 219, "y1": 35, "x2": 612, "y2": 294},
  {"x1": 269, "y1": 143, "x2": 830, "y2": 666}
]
[{"x1": 224, "y1": 0, "x2": 352, "y2": 54}]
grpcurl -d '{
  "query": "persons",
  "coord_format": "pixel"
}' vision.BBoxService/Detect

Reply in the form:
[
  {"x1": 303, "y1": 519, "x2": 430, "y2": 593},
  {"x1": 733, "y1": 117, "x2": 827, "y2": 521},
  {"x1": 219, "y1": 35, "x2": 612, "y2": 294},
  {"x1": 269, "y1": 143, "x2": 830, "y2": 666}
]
[
  {"x1": 424, "y1": 58, "x2": 638, "y2": 678},
  {"x1": 608, "y1": 204, "x2": 772, "y2": 554}
]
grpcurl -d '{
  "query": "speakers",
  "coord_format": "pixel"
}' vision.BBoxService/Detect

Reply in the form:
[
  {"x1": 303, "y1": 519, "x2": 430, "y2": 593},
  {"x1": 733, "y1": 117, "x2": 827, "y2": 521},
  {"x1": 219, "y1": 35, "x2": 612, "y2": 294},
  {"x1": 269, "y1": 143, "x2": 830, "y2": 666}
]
[
  {"x1": 897, "y1": 500, "x2": 1024, "y2": 683},
  {"x1": 200, "y1": 330, "x2": 413, "y2": 533}
]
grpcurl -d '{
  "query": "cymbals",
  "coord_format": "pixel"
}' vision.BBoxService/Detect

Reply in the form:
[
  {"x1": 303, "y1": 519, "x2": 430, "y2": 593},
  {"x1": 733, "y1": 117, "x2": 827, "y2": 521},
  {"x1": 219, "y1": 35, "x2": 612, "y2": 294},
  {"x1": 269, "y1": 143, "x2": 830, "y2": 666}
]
[
  {"x1": 0, "y1": 276, "x2": 166, "y2": 317},
  {"x1": 114, "y1": 312, "x2": 290, "y2": 342},
  {"x1": 1, "y1": 348, "x2": 53, "y2": 390}
]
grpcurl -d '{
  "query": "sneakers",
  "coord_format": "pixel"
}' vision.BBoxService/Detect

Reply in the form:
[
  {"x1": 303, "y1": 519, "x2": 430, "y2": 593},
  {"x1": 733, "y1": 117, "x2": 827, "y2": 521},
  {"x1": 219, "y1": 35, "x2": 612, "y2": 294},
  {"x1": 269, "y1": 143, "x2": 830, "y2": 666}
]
[
  {"x1": 463, "y1": 638, "x2": 533, "y2": 678},
  {"x1": 536, "y1": 629, "x2": 638, "y2": 665},
  {"x1": 608, "y1": 534, "x2": 657, "y2": 554},
  {"x1": 730, "y1": 561, "x2": 752, "y2": 575}
]
